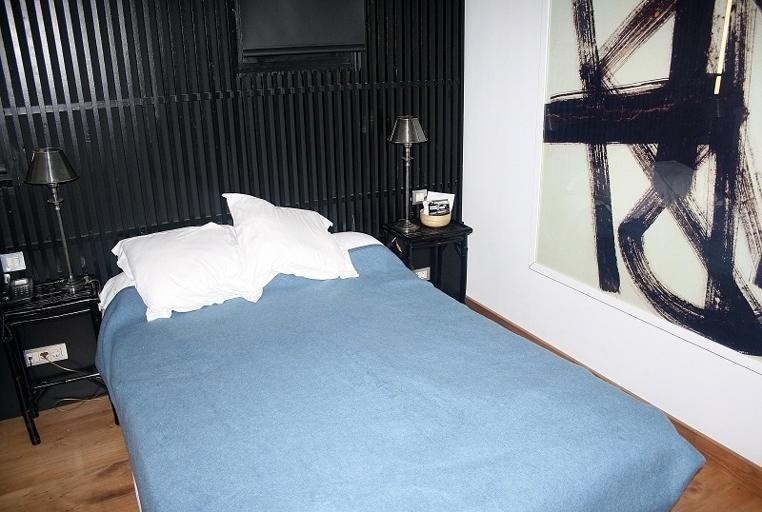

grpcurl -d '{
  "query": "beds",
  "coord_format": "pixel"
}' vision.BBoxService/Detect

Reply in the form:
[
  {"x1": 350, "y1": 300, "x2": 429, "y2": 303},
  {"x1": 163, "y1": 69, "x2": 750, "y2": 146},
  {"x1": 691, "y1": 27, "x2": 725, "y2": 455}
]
[{"x1": 99, "y1": 231, "x2": 707, "y2": 512}]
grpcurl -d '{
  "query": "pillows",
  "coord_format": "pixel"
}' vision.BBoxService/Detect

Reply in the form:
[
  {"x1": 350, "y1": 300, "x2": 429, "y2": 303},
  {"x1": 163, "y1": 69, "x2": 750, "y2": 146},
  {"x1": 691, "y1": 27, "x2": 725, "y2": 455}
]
[{"x1": 111, "y1": 192, "x2": 359, "y2": 322}]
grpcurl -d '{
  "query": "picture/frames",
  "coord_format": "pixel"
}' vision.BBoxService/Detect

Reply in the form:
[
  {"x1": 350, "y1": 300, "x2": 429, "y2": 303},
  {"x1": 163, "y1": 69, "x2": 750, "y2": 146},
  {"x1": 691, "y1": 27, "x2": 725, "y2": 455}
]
[{"x1": 528, "y1": 0, "x2": 762, "y2": 375}]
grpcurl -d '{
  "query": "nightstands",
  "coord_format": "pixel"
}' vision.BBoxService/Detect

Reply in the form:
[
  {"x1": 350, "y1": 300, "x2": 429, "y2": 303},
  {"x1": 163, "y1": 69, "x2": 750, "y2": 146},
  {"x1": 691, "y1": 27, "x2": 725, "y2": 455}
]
[{"x1": 383, "y1": 219, "x2": 473, "y2": 304}]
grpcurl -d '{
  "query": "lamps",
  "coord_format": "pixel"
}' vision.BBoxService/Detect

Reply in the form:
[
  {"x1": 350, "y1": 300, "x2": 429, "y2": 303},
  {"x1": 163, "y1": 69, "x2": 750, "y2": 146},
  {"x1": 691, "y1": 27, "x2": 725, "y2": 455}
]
[
  {"x1": 23, "y1": 149, "x2": 85, "y2": 295},
  {"x1": 389, "y1": 116, "x2": 427, "y2": 234}
]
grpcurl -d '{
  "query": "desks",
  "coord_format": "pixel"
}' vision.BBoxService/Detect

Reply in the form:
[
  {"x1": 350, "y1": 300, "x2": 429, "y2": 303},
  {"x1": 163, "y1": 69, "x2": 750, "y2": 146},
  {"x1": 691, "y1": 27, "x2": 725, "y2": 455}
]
[{"x1": 0, "y1": 274, "x2": 119, "y2": 445}]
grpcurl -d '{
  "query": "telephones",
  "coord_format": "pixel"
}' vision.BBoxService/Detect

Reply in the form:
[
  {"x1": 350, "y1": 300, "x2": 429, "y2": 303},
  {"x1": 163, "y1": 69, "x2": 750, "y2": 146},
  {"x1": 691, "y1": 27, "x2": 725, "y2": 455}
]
[{"x1": 0, "y1": 273, "x2": 36, "y2": 308}]
